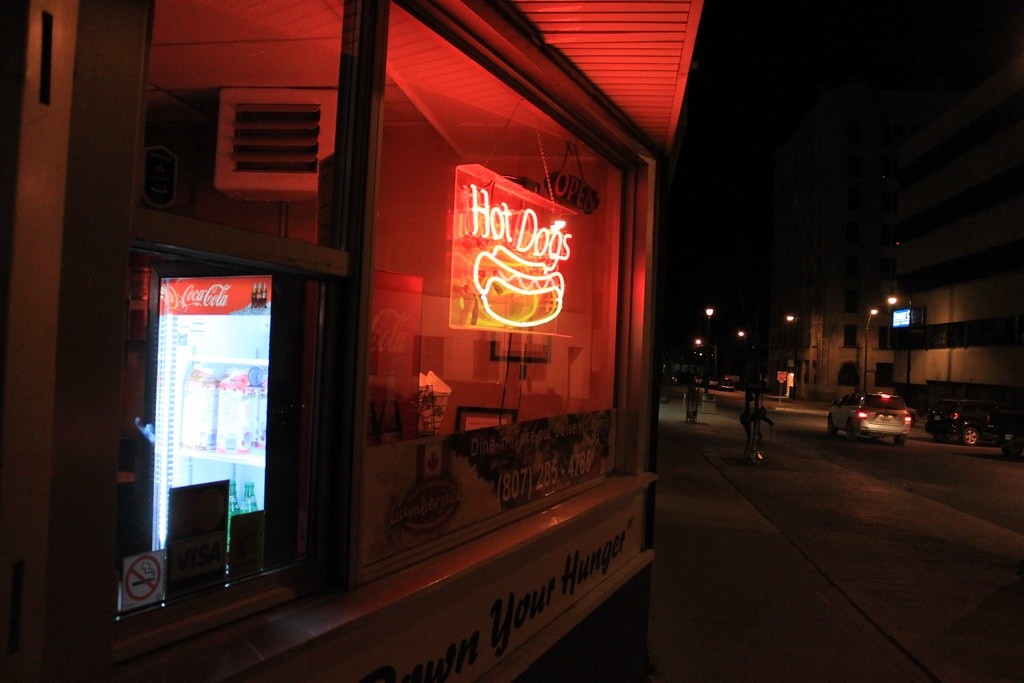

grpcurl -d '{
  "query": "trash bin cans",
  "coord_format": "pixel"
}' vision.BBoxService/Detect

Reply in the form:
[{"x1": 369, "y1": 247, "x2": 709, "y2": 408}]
[{"x1": 701, "y1": 393, "x2": 717, "y2": 414}]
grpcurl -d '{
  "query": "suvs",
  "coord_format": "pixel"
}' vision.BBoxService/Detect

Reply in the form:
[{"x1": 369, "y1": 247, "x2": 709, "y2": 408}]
[
  {"x1": 925, "y1": 398, "x2": 1001, "y2": 444},
  {"x1": 828, "y1": 390, "x2": 912, "y2": 446}
]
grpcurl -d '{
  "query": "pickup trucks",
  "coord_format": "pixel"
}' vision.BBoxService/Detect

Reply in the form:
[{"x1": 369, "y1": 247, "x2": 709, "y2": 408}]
[{"x1": 986, "y1": 398, "x2": 1024, "y2": 458}]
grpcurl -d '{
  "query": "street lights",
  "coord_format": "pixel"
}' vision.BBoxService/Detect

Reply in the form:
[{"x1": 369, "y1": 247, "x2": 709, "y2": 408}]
[
  {"x1": 704, "y1": 306, "x2": 718, "y2": 378},
  {"x1": 736, "y1": 329, "x2": 754, "y2": 390},
  {"x1": 886, "y1": 295, "x2": 913, "y2": 403},
  {"x1": 693, "y1": 337, "x2": 710, "y2": 394},
  {"x1": 784, "y1": 313, "x2": 799, "y2": 399},
  {"x1": 864, "y1": 308, "x2": 879, "y2": 392}
]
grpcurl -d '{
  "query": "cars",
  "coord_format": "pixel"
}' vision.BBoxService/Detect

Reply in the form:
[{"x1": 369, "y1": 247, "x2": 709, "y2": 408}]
[
  {"x1": 694, "y1": 376, "x2": 736, "y2": 391},
  {"x1": 906, "y1": 406, "x2": 917, "y2": 426}
]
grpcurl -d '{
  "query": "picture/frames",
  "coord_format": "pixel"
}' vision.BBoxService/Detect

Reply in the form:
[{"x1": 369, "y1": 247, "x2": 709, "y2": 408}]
[
  {"x1": 490, "y1": 331, "x2": 551, "y2": 363},
  {"x1": 455, "y1": 406, "x2": 518, "y2": 432}
]
